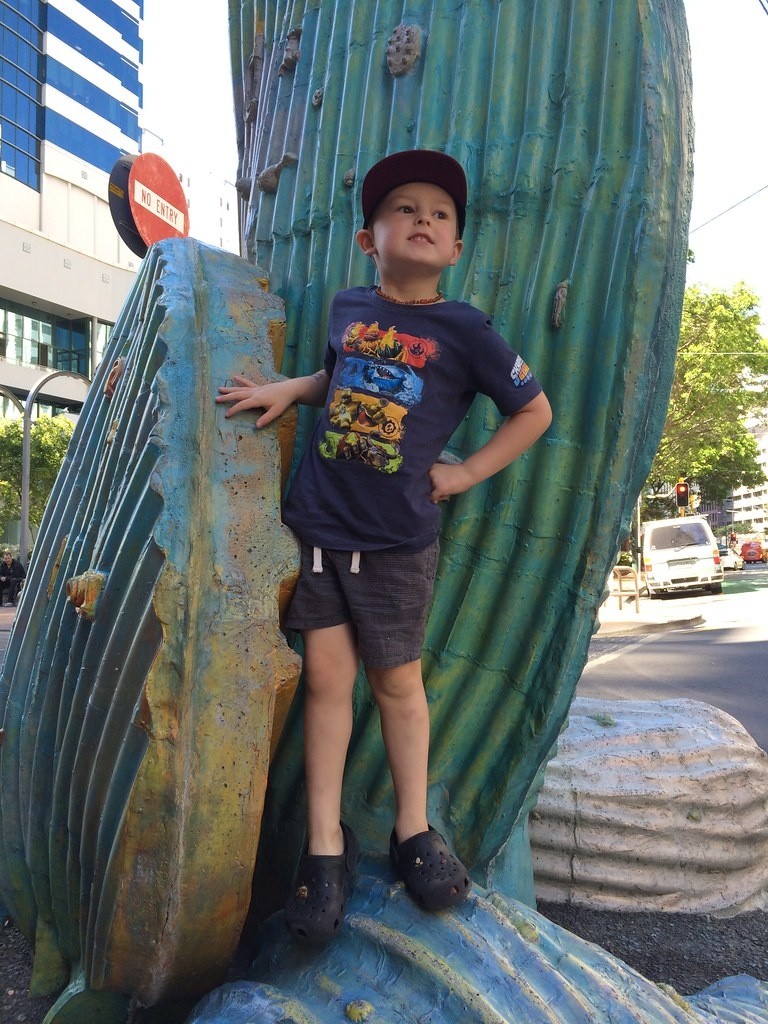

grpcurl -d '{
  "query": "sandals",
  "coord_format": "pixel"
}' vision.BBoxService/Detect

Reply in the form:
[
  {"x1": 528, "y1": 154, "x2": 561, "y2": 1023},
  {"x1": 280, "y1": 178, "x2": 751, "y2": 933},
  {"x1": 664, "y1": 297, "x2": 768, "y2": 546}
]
[
  {"x1": 390, "y1": 825, "x2": 472, "y2": 912},
  {"x1": 286, "y1": 821, "x2": 357, "y2": 942}
]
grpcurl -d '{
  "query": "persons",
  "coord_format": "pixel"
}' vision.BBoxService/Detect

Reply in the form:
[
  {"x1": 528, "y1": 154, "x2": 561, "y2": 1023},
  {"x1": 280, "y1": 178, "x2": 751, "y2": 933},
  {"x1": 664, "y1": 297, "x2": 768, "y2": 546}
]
[
  {"x1": 728, "y1": 531, "x2": 737, "y2": 551},
  {"x1": 0, "y1": 552, "x2": 26, "y2": 606},
  {"x1": 215, "y1": 149, "x2": 552, "y2": 946}
]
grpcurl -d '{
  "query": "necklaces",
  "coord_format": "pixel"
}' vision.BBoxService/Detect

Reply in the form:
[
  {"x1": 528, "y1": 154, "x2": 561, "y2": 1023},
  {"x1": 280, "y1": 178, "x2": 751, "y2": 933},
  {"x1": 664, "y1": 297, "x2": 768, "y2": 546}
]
[{"x1": 376, "y1": 286, "x2": 443, "y2": 305}]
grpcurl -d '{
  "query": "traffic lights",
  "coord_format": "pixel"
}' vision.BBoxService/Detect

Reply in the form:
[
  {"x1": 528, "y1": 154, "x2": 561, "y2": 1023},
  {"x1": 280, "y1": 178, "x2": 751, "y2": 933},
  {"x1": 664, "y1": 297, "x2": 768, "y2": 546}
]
[
  {"x1": 731, "y1": 533, "x2": 738, "y2": 542},
  {"x1": 675, "y1": 482, "x2": 690, "y2": 509}
]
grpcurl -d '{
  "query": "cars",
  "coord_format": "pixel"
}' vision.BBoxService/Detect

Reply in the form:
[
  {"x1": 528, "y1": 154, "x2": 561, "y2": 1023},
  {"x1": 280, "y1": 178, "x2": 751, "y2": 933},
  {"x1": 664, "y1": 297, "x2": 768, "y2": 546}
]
[{"x1": 717, "y1": 548, "x2": 746, "y2": 570}]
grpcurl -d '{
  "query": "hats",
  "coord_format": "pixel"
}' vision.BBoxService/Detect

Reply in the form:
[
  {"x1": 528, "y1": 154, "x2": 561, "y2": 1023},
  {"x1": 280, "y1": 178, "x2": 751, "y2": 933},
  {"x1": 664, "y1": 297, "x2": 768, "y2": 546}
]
[{"x1": 362, "y1": 150, "x2": 467, "y2": 240}]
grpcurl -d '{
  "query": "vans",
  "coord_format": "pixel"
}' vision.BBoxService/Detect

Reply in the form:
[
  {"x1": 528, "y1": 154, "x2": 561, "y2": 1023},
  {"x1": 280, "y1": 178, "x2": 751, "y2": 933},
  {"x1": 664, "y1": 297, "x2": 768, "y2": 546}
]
[
  {"x1": 741, "y1": 542, "x2": 765, "y2": 564},
  {"x1": 643, "y1": 515, "x2": 721, "y2": 599}
]
